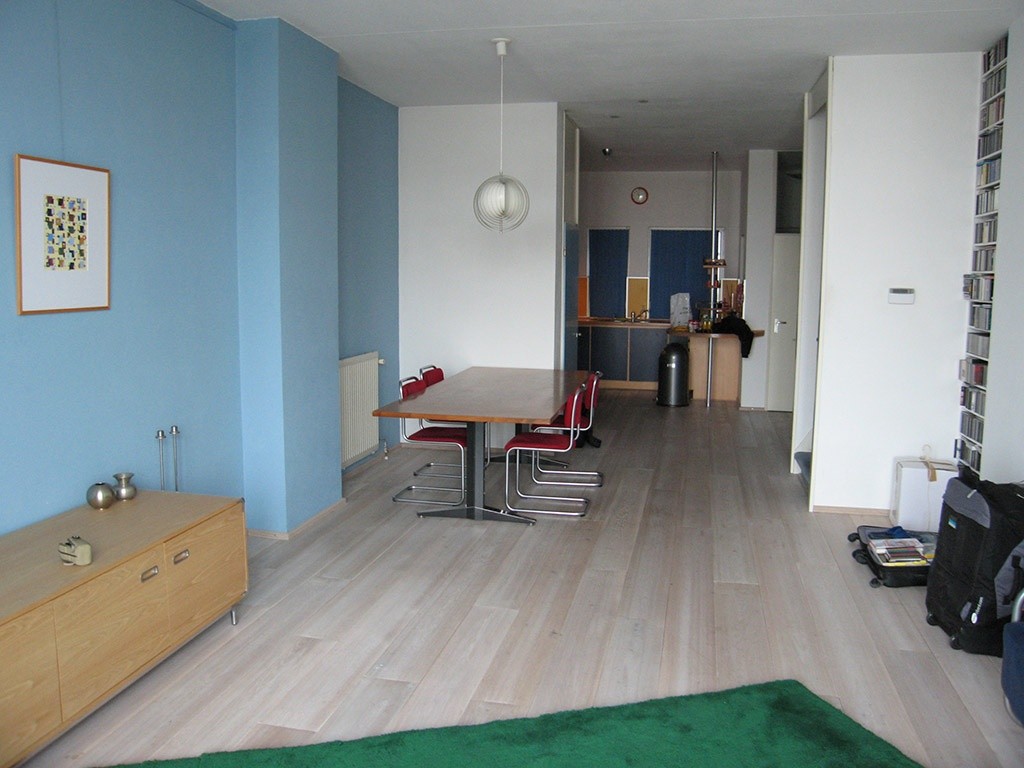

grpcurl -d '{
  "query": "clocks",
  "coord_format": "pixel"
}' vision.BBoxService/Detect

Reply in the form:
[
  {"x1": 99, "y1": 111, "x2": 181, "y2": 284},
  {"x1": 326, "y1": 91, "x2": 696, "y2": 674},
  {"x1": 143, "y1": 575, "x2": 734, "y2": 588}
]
[{"x1": 631, "y1": 187, "x2": 648, "y2": 204}]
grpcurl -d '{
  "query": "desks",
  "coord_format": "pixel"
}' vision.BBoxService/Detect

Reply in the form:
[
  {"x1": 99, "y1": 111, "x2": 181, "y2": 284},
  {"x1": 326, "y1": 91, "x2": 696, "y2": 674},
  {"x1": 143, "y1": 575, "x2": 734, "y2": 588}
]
[
  {"x1": 666, "y1": 327, "x2": 765, "y2": 408},
  {"x1": 372, "y1": 364, "x2": 587, "y2": 526}
]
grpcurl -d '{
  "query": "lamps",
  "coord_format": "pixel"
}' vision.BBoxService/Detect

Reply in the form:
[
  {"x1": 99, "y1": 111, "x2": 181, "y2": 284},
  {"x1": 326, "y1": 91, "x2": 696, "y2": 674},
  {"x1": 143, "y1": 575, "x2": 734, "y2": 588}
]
[{"x1": 470, "y1": 38, "x2": 530, "y2": 237}]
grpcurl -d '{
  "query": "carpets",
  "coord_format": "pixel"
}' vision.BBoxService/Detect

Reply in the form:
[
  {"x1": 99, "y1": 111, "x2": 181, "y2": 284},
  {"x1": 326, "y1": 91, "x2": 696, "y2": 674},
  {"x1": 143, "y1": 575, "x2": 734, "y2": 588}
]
[{"x1": 128, "y1": 679, "x2": 922, "y2": 768}]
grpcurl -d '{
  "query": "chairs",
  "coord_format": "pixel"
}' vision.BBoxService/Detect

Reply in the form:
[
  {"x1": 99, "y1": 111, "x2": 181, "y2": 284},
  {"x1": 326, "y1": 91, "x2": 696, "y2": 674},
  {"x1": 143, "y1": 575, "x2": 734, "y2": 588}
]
[
  {"x1": 392, "y1": 377, "x2": 466, "y2": 506},
  {"x1": 503, "y1": 384, "x2": 590, "y2": 516},
  {"x1": 414, "y1": 365, "x2": 490, "y2": 478},
  {"x1": 530, "y1": 371, "x2": 603, "y2": 487}
]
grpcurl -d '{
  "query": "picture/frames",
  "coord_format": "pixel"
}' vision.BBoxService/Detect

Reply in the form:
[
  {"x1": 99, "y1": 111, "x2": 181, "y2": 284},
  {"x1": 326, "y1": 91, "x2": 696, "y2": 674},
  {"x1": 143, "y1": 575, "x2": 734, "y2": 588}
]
[{"x1": 14, "y1": 153, "x2": 111, "y2": 315}]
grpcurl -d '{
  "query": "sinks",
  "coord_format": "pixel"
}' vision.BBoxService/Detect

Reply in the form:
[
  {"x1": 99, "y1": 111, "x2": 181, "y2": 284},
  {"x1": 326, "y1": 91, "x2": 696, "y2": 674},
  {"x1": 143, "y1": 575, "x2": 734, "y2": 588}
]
[{"x1": 626, "y1": 319, "x2": 670, "y2": 324}]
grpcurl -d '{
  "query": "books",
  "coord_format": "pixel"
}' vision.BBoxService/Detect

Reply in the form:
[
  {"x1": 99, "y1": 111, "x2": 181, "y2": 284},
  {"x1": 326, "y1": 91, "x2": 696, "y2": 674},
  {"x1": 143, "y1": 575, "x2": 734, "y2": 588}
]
[{"x1": 869, "y1": 34, "x2": 1008, "y2": 562}]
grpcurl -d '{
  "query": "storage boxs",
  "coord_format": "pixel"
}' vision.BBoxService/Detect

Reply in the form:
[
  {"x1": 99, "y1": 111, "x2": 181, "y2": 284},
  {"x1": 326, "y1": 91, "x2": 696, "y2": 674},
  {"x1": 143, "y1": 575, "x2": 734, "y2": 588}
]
[{"x1": 890, "y1": 458, "x2": 959, "y2": 532}]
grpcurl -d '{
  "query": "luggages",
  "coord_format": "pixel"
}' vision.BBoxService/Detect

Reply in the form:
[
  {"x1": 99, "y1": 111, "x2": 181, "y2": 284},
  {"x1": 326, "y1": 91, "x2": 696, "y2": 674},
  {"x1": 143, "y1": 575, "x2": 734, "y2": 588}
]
[
  {"x1": 848, "y1": 522, "x2": 933, "y2": 588},
  {"x1": 927, "y1": 477, "x2": 1024, "y2": 650}
]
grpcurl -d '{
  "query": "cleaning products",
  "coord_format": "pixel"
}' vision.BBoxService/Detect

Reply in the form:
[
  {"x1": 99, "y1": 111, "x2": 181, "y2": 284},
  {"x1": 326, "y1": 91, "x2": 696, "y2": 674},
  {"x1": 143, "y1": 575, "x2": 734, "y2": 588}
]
[{"x1": 640, "y1": 304, "x2": 648, "y2": 320}]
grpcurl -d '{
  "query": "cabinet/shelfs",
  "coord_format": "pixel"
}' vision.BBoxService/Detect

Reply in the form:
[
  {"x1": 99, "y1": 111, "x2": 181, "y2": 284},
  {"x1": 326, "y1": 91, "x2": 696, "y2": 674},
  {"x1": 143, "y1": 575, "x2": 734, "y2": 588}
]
[
  {"x1": 0, "y1": 493, "x2": 248, "y2": 768},
  {"x1": 576, "y1": 326, "x2": 686, "y2": 390},
  {"x1": 959, "y1": 29, "x2": 1009, "y2": 473}
]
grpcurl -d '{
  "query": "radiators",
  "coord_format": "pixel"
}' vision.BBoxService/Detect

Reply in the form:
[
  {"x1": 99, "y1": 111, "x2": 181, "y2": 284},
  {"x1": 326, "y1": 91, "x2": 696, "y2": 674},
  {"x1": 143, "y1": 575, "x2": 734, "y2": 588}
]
[{"x1": 339, "y1": 350, "x2": 390, "y2": 471}]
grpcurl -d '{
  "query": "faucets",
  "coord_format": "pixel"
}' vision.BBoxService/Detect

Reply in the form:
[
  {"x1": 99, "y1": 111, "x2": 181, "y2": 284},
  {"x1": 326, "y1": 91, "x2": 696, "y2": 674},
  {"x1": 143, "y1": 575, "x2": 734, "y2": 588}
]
[{"x1": 631, "y1": 309, "x2": 648, "y2": 322}]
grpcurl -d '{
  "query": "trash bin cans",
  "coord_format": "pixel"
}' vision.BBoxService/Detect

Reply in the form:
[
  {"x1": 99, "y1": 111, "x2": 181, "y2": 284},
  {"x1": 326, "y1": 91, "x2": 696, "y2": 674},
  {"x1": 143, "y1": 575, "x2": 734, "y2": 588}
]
[{"x1": 655, "y1": 343, "x2": 689, "y2": 407}]
